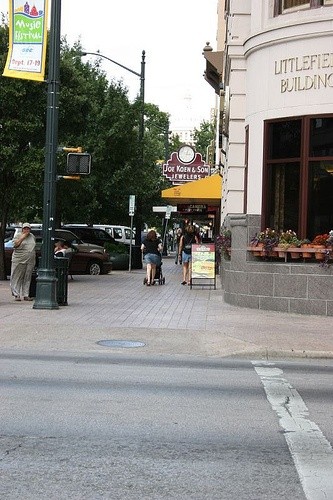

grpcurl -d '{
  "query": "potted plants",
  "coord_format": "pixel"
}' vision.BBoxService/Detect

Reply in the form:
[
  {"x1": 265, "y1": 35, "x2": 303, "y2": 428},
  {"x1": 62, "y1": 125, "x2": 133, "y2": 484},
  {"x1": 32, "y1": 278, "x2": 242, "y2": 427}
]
[
  {"x1": 289, "y1": 237, "x2": 300, "y2": 258},
  {"x1": 277, "y1": 238, "x2": 290, "y2": 258},
  {"x1": 300, "y1": 239, "x2": 313, "y2": 258}
]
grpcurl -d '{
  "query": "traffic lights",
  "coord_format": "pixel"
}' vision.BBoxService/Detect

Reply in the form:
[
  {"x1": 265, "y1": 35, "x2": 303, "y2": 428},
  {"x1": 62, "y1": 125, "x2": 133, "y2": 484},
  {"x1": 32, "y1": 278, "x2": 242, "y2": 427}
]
[{"x1": 67, "y1": 152, "x2": 90, "y2": 174}]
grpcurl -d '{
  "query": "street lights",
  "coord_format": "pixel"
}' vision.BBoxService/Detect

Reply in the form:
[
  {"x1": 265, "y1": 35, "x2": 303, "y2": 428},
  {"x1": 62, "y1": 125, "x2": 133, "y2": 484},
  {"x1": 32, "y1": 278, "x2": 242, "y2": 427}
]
[
  {"x1": 63, "y1": 49, "x2": 147, "y2": 269},
  {"x1": 144, "y1": 114, "x2": 170, "y2": 257}
]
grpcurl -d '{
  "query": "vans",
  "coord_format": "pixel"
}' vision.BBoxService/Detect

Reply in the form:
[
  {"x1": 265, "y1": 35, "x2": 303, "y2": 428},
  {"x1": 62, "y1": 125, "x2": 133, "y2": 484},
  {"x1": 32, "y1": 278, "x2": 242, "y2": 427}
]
[{"x1": 66, "y1": 222, "x2": 136, "y2": 246}]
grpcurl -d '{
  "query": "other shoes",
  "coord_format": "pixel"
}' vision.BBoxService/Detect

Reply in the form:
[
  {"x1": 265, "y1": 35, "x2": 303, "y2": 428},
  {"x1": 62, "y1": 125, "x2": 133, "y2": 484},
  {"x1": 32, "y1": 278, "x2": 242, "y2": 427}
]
[
  {"x1": 151, "y1": 280, "x2": 155, "y2": 285},
  {"x1": 24, "y1": 297, "x2": 33, "y2": 301},
  {"x1": 147, "y1": 281, "x2": 150, "y2": 285},
  {"x1": 181, "y1": 281, "x2": 186, "y2": 285},
  {"x1": 15, "y1": 296, "x2": 21, "y2": 301}
]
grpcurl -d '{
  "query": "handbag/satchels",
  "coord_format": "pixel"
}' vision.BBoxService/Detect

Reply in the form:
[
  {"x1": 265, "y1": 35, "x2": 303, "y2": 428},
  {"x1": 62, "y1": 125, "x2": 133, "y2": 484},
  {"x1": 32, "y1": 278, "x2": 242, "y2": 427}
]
[{"x1": 184, "y1": 247, "x2": 191, "y2": 254}]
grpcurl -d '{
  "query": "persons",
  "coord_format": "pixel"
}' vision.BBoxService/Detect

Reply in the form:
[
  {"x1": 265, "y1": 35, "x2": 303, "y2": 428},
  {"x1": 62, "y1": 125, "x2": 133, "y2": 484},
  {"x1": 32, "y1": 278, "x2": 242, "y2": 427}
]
[
  {"x1": 11, "y1": 223, "x2": 36, "y2": 302},
  {"x1": 141, "y1": 230, "x2": 162, "y2": 285},
  {"x1": 166, "y1": 225, "x2": 201, "y2": 286},
  {"x1": 54, "y1": 241, "x2": 75, "y2": 257}
]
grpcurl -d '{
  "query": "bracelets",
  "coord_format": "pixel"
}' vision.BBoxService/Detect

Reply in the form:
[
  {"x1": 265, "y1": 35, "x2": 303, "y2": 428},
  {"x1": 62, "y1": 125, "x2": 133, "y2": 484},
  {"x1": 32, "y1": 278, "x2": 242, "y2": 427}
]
[{"x1": 178, "y1": 254, "x2": 181, "y2": 256}]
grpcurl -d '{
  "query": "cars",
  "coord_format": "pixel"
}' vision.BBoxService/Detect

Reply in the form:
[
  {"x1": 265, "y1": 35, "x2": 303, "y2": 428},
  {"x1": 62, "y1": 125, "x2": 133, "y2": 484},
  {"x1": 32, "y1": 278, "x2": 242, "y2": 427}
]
[{"x1": 3, "y1": 236, "x2": 112, "y2": 276}]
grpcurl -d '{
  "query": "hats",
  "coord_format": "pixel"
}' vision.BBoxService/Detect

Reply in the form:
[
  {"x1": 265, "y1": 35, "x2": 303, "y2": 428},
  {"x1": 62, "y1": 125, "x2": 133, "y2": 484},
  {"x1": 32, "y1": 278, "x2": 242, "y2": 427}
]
[{"x1": 22, "y1": 222, "x2": 31, "y2": 229}]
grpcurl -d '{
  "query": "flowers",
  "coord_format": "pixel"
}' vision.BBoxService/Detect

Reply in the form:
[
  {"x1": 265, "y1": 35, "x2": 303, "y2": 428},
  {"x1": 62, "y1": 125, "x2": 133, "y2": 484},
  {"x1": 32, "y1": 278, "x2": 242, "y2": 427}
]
[
  {"x1": 251, "y1": 228, "x2": 279, "y2": 256},
  {"x1": 311, "y1": 234, "x2": 329, "y2": 245},
  {"x1": 326, "y1": 229, "x2": 333, "y2": 246}
]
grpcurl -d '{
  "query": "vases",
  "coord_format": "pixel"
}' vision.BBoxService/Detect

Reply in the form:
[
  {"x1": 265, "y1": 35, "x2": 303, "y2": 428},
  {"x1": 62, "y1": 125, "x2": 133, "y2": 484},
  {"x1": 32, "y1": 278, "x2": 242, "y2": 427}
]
[
  {"x1": 326, "y1": 245, "x2": 333, "y2": 259},
  {"x1": 314, "y1": 245, "x2": 325, "y2": 259},
  {"x1": 253, "y1": 241, "x2": 277, "y2": 257}
]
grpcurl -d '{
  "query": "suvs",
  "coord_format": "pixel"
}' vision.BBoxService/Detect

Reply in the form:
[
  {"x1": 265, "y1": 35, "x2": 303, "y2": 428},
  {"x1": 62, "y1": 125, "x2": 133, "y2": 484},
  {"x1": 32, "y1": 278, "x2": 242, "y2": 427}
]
[
  {"x1": 61, "y1": 226, "x2": 140, "y2": 264},
  {"x1": 25, "y1": 228, "x2": 108, "y2": 253}
]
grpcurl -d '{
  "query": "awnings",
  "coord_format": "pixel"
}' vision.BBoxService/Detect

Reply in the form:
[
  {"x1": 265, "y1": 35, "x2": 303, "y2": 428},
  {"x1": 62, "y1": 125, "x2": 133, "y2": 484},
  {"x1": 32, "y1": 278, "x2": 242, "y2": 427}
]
[{"x1": 162, "y1": 174, "x2": 222, "y2": 205}]
